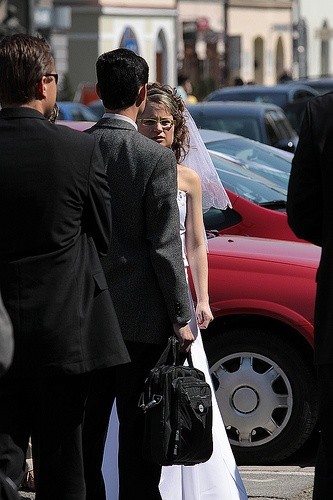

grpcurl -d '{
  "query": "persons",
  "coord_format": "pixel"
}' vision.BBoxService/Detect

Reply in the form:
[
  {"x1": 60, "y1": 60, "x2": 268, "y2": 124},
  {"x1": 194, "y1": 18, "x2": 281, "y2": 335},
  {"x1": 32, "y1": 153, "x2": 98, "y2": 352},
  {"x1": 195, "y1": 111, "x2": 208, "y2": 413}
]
[
  {"x1": 101, "y1": 83, "x2": 215, "y2": 500},
  {"x1": 83, "y1": 48, "x2": 195, "y2": 500},
  {"x1": 1, "y1": 30, "x2": 131, "y2": 500},
  {"x1": 285, "y1": 90, "x2": 333, "y2": 500},
  {"x1": 181, "y1": 76, "x2": 198, "y2": 102}
]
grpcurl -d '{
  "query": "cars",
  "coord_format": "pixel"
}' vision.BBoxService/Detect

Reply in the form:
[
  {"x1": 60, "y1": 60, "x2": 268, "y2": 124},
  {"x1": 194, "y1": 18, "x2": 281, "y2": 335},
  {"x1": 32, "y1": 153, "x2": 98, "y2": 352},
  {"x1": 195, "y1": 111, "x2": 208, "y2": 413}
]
[
  {"x1": 186, "y1": 102, "x2": 299, "y2": 154},
  {"x1": 285, "y1": 78, "x2": 333, "y2": 115},
  {"x1": 202, "y1": 87, "x2": 319, "y2": 134},
  {"x1": 54, "y1": 101, "x2": 100, "y2": 122},
  {"x1": 48, "y1": 121, "x2": 322, "y2": 466},
  {"x1": 182, "y1": 129, "x2": 294, "y2": 210}
]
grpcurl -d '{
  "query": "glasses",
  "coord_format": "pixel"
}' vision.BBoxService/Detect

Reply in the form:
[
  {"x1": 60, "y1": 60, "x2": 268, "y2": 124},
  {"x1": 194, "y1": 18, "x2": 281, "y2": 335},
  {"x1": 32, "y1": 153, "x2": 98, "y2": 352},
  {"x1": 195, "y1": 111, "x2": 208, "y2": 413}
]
[
  {"x1": 43, "y1": 73, "x2": 59, "y2": 85},
  {"x1": 137, "y1": 118, "x2": 176, "y2": 130}
]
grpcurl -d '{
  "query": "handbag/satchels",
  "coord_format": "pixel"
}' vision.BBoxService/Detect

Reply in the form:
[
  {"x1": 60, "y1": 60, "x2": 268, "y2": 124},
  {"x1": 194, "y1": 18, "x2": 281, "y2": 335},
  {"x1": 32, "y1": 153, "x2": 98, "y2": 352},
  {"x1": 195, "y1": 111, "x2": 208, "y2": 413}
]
[{"x1": 136, "y1": 333, "x2": 213, "y2": 466}]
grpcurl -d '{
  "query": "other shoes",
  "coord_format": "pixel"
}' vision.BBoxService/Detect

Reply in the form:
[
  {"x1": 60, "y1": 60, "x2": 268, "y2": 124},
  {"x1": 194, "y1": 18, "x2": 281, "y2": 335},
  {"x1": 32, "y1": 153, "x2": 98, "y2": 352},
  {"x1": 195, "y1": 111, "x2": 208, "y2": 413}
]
[{"x1": 22, "y1": 470, "x2": 36, "y2": 491}]
